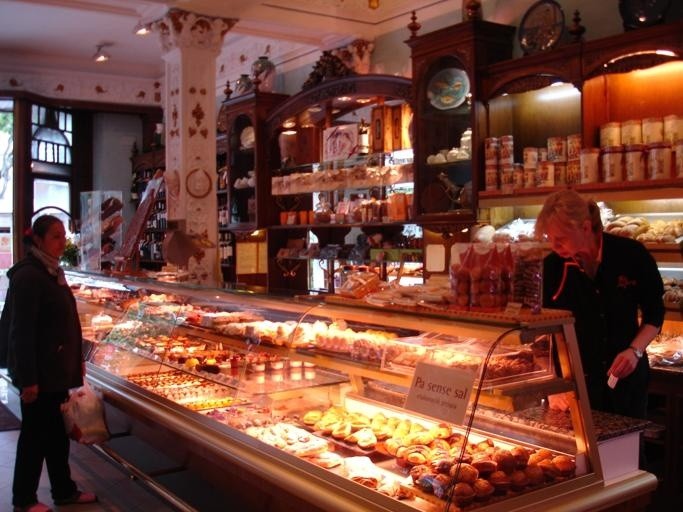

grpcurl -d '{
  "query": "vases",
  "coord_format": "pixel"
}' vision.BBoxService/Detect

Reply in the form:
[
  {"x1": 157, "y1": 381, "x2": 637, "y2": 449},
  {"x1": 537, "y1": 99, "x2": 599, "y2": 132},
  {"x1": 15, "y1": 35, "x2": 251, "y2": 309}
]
[
  {"x1": 235, "y1": 73, "x2": 253, "y2": 95},
  {"x1": 249, "y1": 55, "x2": 275, "y2": 93}
]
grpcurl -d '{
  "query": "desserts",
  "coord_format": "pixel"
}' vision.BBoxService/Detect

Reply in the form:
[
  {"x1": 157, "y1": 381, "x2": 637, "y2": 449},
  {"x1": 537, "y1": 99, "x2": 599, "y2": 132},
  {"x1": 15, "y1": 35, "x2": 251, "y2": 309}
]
[{"x1": 74, "y1": 285, "x2": 532, "y2": 458}]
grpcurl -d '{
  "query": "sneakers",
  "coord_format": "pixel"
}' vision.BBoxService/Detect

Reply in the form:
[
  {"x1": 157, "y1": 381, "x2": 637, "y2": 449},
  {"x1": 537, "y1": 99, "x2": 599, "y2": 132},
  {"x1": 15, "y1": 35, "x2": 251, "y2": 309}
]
[
  {"x1": 54, "y1": 492, "x2": 98, "y2": 504},
  {"x1": 14, "y1": 503, "x2": 53, "y2": 512}
]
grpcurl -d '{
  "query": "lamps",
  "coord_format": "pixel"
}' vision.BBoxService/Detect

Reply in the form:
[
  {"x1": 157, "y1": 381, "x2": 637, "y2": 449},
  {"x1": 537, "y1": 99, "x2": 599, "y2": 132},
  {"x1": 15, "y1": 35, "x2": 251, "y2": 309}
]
[
  {"x1": 90, "y1": 42, "x2": 113, "y2": 62},
  {"x1": 131, "y1": 21, "x2": 153, "y2": 35}
]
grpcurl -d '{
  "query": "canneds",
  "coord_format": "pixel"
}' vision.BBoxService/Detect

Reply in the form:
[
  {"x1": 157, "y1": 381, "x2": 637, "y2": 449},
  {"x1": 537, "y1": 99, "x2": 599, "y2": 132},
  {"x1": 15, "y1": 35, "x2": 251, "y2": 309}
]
[{"x1": 485, "y1": 115, "x2": 683, "y2": 190}]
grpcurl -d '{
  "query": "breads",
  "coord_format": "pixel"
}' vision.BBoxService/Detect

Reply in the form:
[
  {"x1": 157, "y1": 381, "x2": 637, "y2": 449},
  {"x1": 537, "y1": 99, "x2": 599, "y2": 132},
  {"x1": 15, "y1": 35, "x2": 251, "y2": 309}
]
[
  {"x1": 341, "y1": 261, "x2": 543, "y2": 308},
  {"x1": 156, "y1": 269, "x2": 198, "y2": 285},
  {"x1": 604, "y1": 215, "x2": 683, "y2": 245},
  {"x1": 302, "y1": 406, "x2": 576, "y2": 503},
  {"x1": 663, "y1": 278, "x2": 683, "y2": 303}
]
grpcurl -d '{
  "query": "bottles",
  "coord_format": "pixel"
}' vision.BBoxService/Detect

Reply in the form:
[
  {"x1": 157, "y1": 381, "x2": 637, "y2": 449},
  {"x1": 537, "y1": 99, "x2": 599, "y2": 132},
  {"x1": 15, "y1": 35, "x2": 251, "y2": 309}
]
[
  {"x1": 357, "y1": 117, "x2": 368, "y2": 156},
  {"x1": 358, "y1": 200, "x2": 383, "y2": 225},
  {"x1": 126, "y1": 175, "x2": 234, "y2": 270}
]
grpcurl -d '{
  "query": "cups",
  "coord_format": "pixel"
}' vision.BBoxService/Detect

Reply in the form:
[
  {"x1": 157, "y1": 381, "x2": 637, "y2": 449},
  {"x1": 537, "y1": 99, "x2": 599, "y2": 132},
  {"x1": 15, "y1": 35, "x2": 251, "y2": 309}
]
[{"x1": 298, "y1": 209, "x2": 314, "y2": 224}]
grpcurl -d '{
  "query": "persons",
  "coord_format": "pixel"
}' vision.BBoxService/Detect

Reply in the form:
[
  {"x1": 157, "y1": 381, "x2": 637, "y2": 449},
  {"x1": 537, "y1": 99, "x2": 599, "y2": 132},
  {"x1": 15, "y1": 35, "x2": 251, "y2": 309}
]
[
  {"x1": 534, "y1": 191, "x2": 666, "y2": 421},
  {"x1": 0, "y1": 214, "x2": 97, "y2": 512}
]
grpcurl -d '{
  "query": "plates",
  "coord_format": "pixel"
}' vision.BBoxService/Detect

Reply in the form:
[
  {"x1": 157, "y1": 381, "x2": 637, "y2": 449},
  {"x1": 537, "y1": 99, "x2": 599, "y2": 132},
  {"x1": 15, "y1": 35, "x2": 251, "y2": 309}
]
[
  {"x1": 426, "y1": 68, "x2": 467, "y2": 110},
  {"x1": 611, "y1": 1, "x2": 664, "y2": 30},
  {"x1": 516, "y1": 2, "x2": 564, "y2": 55}
]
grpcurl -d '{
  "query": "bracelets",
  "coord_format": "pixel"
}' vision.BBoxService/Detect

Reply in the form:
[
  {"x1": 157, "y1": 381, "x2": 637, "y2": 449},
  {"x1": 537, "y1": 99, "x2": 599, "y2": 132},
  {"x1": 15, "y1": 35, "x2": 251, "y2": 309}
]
[{"x1": 629, "y1": 344, "x2": 643, "y2": 360}]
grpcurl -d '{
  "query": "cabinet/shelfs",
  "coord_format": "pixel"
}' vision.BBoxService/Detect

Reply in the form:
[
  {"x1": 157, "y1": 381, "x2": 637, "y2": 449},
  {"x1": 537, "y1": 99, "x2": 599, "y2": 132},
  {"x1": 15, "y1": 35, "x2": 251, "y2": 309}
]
[
  {"x1": 400, "y1": 17, "x2": 518, "y2": 217},
  {"x1": 261, "y1": 71, "x2": 422, "y2": 294},
  {"x1": 128, "y1": 86, "x2": 289, "y2": 289},
  {"x1": 477, "y1": 41, "x2": 582, "y2": 243},
  {"x1": 63, "y1": 266, "x2": 603, "y2": 511},
  {"x1": 581, "y1": 18, "x2": 682, "y2": 376}
]
